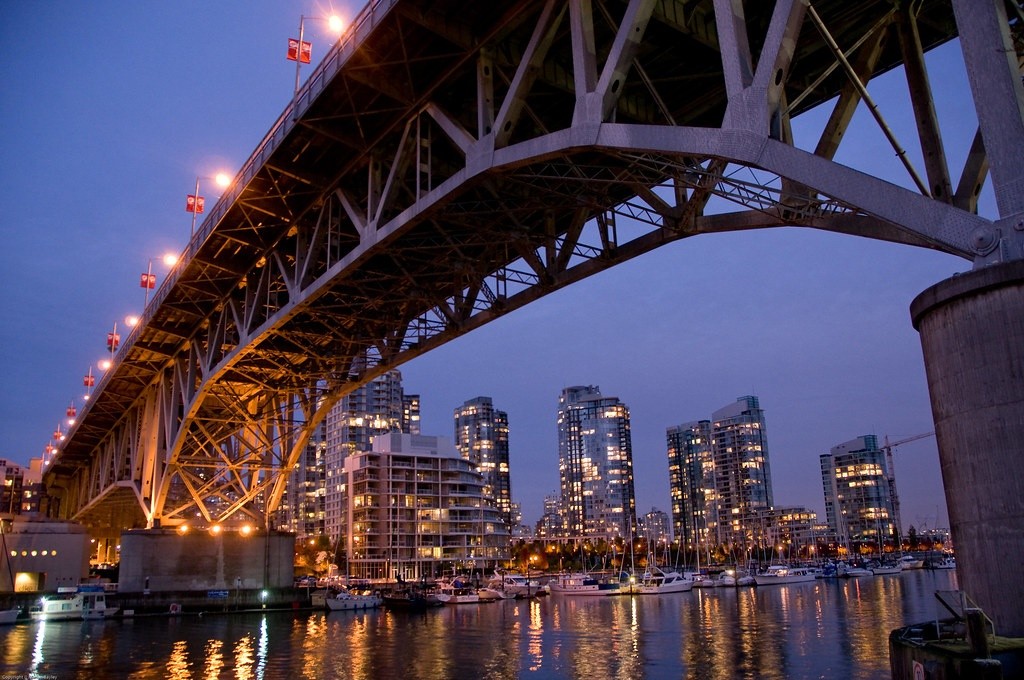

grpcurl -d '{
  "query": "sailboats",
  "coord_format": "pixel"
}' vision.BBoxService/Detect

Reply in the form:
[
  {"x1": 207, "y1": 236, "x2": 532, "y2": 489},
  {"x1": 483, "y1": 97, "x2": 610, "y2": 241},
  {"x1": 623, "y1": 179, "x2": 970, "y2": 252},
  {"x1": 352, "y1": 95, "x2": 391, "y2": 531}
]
[{"x1": 320, "y1": 493, "x2": 959, "y2": 608}]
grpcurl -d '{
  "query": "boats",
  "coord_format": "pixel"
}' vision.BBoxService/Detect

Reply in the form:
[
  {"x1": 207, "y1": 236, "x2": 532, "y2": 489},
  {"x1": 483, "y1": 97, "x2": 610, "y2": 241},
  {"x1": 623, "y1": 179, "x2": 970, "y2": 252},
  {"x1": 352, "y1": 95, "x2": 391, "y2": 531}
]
[{"x1": 29, "y1": 587, "x2": 121, "y2": 621}]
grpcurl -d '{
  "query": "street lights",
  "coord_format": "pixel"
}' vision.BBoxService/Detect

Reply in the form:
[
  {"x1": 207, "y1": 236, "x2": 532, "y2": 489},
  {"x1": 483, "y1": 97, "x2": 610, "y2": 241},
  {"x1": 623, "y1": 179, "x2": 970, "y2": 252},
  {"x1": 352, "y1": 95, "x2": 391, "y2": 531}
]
[
  {"x1": 192, "y1": 172, "x2": 231, "y2": 238},
  {"x1": 43, "y1": 360, "x2": 110, "y2": 465},
  {"x1": 294, "y1": 15, "x2": 343, "y2": 92},
  {"x1": 111, "y1": 316, "x2": 139, "y2": 364},
  {"x1": 146, "y1": 256, "x2": 177, "y2": 306}
]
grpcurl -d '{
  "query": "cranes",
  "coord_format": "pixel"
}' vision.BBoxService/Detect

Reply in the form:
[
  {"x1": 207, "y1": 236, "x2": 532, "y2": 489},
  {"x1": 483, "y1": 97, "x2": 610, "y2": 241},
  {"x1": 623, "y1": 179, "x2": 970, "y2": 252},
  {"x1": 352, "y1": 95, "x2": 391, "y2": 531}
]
[{"x1": 880, "y1": 430, "x2": 936, "y2": 547}]
